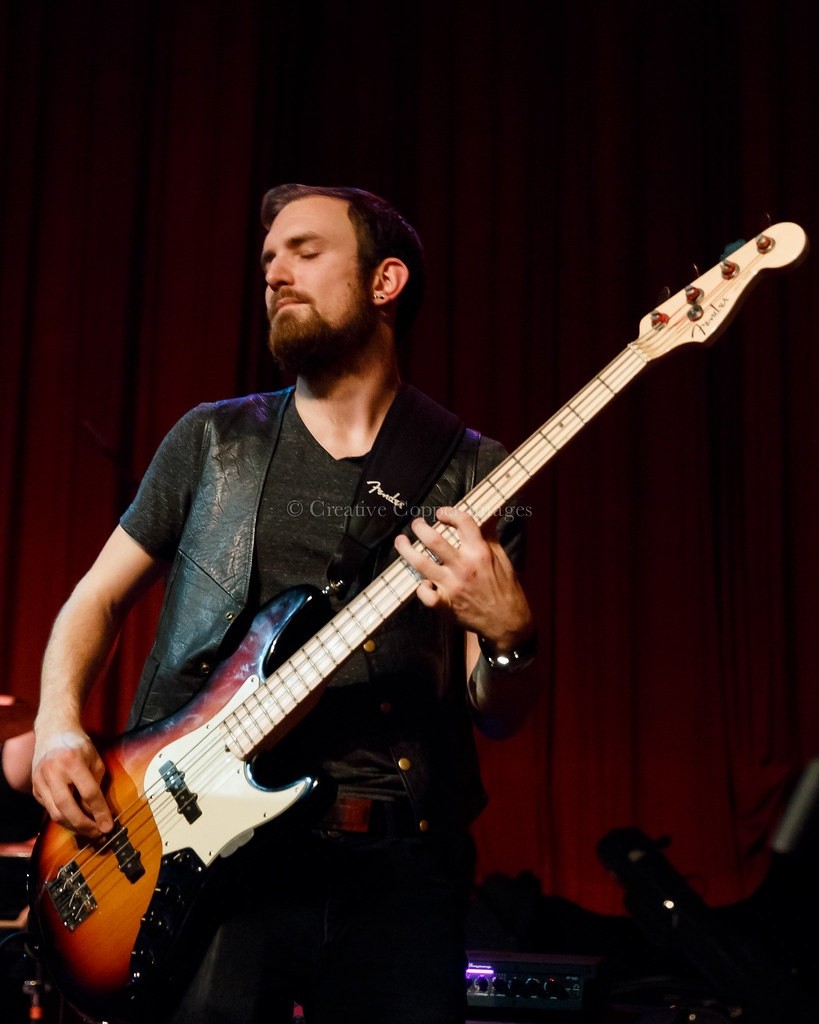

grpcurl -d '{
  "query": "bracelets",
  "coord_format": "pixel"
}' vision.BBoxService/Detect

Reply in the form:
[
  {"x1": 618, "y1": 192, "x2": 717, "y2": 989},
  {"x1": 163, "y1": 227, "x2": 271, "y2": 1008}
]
[{"x1": 480, "y1": 631, "x2": 543, "y2": 671}]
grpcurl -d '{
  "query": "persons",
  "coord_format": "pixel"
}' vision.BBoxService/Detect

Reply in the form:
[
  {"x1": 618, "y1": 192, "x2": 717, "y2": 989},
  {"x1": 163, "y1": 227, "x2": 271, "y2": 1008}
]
[
  {"x1": 0, "y1": 730, "x2": 35, "y2": 813},
  {"x1": 29, "y1": 179, "x2": 542, "y2": 1024}
]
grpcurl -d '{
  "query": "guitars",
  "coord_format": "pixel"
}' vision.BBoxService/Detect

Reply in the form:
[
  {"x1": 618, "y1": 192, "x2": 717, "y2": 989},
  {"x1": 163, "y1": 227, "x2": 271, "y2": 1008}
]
[{"x1": 26, "y1": 209, "x2": 811, "y2": 1024}]
